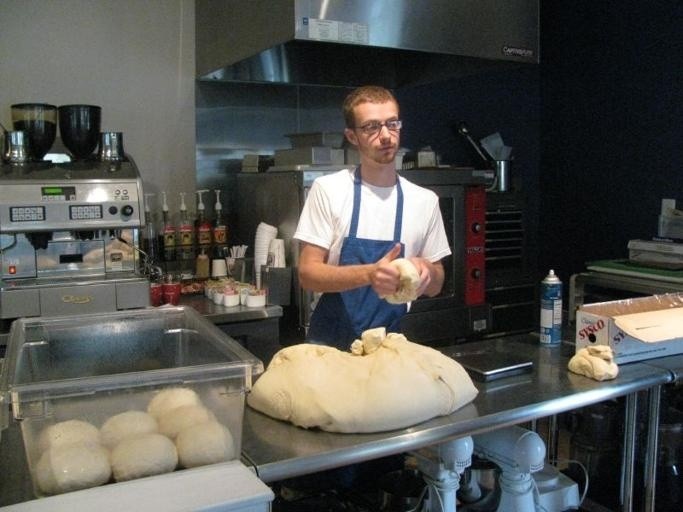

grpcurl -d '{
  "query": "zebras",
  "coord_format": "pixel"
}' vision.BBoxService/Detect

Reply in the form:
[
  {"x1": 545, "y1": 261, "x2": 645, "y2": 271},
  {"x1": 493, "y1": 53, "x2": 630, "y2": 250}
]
[{"x1": 304, "y1": 186, "x2": 489, "y2": 342}]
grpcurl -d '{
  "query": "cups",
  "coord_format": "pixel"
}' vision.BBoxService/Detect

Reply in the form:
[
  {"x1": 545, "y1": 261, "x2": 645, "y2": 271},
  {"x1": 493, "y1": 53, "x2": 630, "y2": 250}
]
[{"x1": 353, "y1": 119, "x2": 403, "y2": 133}]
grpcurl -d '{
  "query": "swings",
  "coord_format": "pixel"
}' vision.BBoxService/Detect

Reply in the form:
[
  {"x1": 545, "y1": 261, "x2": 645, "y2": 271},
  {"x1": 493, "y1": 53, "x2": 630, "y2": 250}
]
[{"x1": 404, "y1": 422, "x2": 590, "y2": 512}]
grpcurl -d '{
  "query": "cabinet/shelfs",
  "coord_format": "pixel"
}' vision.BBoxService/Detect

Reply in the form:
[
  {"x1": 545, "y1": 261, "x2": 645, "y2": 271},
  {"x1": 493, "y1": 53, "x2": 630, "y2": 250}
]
[{"x1": 196, "y1": 248, "x2": 211, "y2": 278}]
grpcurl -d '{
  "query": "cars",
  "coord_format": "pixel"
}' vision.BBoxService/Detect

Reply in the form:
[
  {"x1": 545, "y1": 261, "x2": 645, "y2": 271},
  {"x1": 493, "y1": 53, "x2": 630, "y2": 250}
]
[
  {"x1": 145, "y1": 271, "x2": 181, "y2": 307},
  {"x1": 203, "y1": 279, "x2": 267, "y2": 308},
  {"x1": 266, "y1": 239, "x2": 286, "y2": 270},
  {"x1": 210, "y1": 260, "x2": 228, "y2": 277}
]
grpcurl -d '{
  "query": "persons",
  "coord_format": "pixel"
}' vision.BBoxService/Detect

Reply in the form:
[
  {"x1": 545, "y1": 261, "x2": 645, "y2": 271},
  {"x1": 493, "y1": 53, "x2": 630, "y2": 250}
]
[{"x1": 292, "y1": 84, "x2": 453, "y2": 355}]
